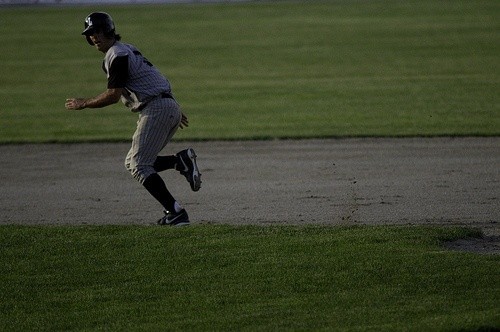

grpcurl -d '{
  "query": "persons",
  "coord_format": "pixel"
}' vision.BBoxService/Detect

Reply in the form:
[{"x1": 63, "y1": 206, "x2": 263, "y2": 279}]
[{"x1": 65, "y1": 10, "x2": 202, "y2": 226}]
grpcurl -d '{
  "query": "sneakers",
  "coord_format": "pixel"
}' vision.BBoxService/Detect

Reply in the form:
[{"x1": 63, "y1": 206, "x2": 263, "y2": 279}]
[
  {"x1": 176, "y1": 148, "x2": 202, "y2": 192},
  {"x1": 154, "y1": 208, "x2": 191, "y2": 225}
]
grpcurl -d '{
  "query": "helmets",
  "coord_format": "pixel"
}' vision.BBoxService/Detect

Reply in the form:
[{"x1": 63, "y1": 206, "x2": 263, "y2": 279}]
[{"x1": 81, "y1": 11, "x2": 115, "y2": 46}]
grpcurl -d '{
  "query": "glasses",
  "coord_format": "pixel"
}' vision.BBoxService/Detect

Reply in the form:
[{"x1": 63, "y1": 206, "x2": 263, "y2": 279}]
[{"x1": 87, "y1": 29, "x2": 101, "y2": 36}]
